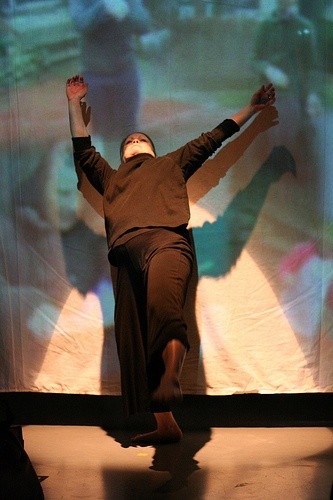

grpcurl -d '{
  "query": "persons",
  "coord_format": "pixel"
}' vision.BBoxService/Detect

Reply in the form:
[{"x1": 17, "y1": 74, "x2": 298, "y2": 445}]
[{"x1": 62, "y1": 73, "x2": 276, "y2": 449}]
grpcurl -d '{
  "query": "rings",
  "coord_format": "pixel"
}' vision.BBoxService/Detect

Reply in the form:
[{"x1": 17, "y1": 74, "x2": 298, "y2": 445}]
[{"x1": 268, "y1": 95, "x2": 273, "y2": 99}]
